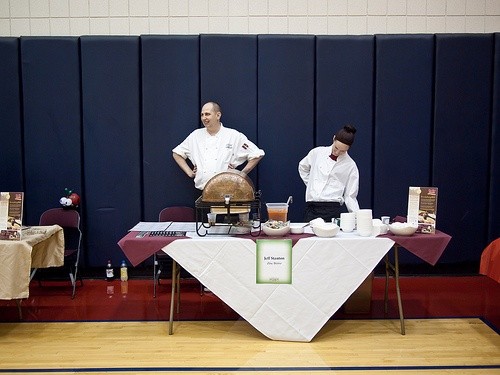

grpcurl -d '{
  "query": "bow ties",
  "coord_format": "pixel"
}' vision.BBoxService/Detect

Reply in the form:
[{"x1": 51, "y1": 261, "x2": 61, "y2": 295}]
[{"x1": 329, "y1": 153, "x2": 338, "y2": 161}]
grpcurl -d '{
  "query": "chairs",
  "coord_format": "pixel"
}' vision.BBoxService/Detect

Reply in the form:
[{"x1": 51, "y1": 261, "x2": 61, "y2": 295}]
[
  {"x1": 154, "y1": 207, "x2": 202, "y2": 298},
  {"x1": 39, "y1": 208, "x2": 81, "y2": 300}
]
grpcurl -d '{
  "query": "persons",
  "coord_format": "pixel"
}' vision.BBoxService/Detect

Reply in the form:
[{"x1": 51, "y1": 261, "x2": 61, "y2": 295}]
[
  {"x1": 172, "y1": 101, "x2": 265, "y2": 293},
  {"x1": 297, "y1": 124, "x2": 360, "y2": 222}
]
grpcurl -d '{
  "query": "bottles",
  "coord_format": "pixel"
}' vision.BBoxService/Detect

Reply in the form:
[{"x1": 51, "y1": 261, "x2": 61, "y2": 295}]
[
  {"x1": 121, "y1": 260, "x2": 128, "y2": 281},
  {"x1": 106, "y1": 261, "x2": 114, "y2": 281},
  {"x1": 121, "y1": 281, "x2": 128, "y2": 298},
  {"x1": 106, "y1": 284, "x2": 115, "y2": 298}
]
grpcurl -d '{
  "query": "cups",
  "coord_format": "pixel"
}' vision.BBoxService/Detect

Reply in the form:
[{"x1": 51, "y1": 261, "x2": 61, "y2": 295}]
[
  {"x1": 340, "y1": 213, "x2": 355, "y2": 232},
  {"x1": 239, "y1": 213, "x2": 249, "y2": 223},
  {"x1": 357, "y1": 209, "x2": 372, "y2": 237},
  {"x1": 381, "y1": 217, "x2": 390, "y2": 224},
  {"x1": 207, "y1": 213, "x2": 216, "y2": 225},
  {"x1": 332, "y1": 218, "x2": 340, "y2": 227}
]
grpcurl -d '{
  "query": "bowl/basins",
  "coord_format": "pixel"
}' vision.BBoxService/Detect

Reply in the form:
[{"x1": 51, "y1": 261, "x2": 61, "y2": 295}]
[
  {"x1": 262, "y1": 219, "x2": 290, "y2": 236},
  {"x1": 387, "y1": 222, "x2": 418, "y2": 236},
  {"x1": 372, "y1": 219, "x2": 388, "y2": 235},
  {"x1": 312, "y1": 223, "x2": 340, "y2": 237},
  {"x1": 290, "y1": 227, "x2": 304, "y2": 234}
]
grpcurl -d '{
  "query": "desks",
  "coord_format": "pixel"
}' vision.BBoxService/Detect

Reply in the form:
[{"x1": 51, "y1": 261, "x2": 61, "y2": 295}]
[
  {"x1": 118, "y1": 222, "x2": 452, "y2": 342},
  {"x1": 0, "y1": 224, "x2": 65, "y2": 320}
]
